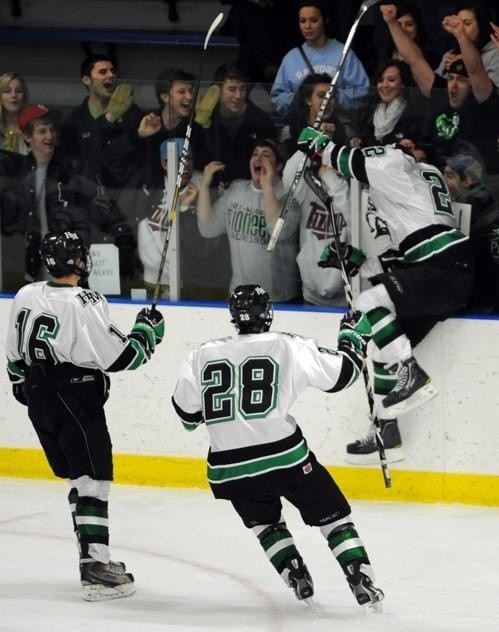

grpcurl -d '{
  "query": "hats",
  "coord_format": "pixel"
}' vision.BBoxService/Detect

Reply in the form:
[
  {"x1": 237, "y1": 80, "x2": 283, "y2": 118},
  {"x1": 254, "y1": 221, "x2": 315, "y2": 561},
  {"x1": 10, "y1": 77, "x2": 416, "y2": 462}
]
[
  {"x1": 19, "y1": 103, "x2": 63, "y2": 131},
  {"x1": 448, "y1": 59, "x2": 470, "y2": 78}
]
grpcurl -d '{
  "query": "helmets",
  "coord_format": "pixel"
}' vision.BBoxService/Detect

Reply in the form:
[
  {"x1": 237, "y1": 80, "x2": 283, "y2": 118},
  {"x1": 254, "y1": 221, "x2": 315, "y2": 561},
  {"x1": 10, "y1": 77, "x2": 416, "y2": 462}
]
[
  {"x1": 230, "y1": 285, "x2": 274, "y2": 331},
  {"x1": 39, "y1": 231, "x2": 92, "y2": 281}
]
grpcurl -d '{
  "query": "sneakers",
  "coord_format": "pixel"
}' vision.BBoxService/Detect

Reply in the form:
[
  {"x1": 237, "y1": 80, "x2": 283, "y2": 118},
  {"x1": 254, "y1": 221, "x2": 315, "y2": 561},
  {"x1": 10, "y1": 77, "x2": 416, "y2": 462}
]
[
  {"x1": 382, "y1": 356, "x2": 432, "y2": 408},
  {"x1": 347, "y1": 417, "x2": 402, "y2": 453},
  {"x1": 81, "y1": 537, "x2": 134, "y2": 587},
  {"x1": 287, "y1": 556, "x2": 313, "y2": 600},
  {"x1": 343, "y1": 561, "x2": 385, "y2": 605}
]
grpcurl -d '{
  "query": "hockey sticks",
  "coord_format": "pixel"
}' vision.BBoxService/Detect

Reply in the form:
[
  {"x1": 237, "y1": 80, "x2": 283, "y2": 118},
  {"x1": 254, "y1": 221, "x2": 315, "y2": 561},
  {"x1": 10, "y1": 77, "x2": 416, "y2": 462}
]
[
  {"x1": 304, "y1": 154, "x2": 392, "y2": 488},
  {"x1": 152, "y1": 13, "x2": 224, "y2": 311},
  {"x1": 267, "y1": 0, "x2": 379, "y2": 251}
]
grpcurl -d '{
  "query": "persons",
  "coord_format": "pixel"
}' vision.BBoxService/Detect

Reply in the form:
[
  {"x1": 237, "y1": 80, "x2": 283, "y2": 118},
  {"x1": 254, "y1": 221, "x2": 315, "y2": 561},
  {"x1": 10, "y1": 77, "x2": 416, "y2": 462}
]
[
  {"x1": 296, "y1": 125, "x2": 473, "y2": 457},
  {"x1": 4, "y1": 230, "x2": 166, "y2": 587},
  {"x1": 1, "y1": 1, "x2": 498, "y2": 315},
  {"x1": 170, "y1": 284, "x2": 385, "y2": 607}
]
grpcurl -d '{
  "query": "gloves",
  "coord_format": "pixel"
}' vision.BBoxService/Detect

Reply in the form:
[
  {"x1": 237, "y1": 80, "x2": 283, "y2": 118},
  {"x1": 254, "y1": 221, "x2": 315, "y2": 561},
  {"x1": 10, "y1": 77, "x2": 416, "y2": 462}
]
[
  {"x1": 297, "y1": 126, "x2": 330, "y2": 157},
  {"x1": 6, "y1": 361, "x2": 27, "y2": 406},
  {"x1": 317, "y1": 240, "x2": 365, "y2": 277},
  {"x1": 195, "y1": 84, "x2": 222, "y2": 124},
  {"x1": 104, "y1": 84, "x2": 134, "y2": 122},
  {"x1": 338, "y1": 310, "x2": 374, "y2": 358},
  {"x1": 131, "y1": 307, "x2": 164, "y2": 353}
]
